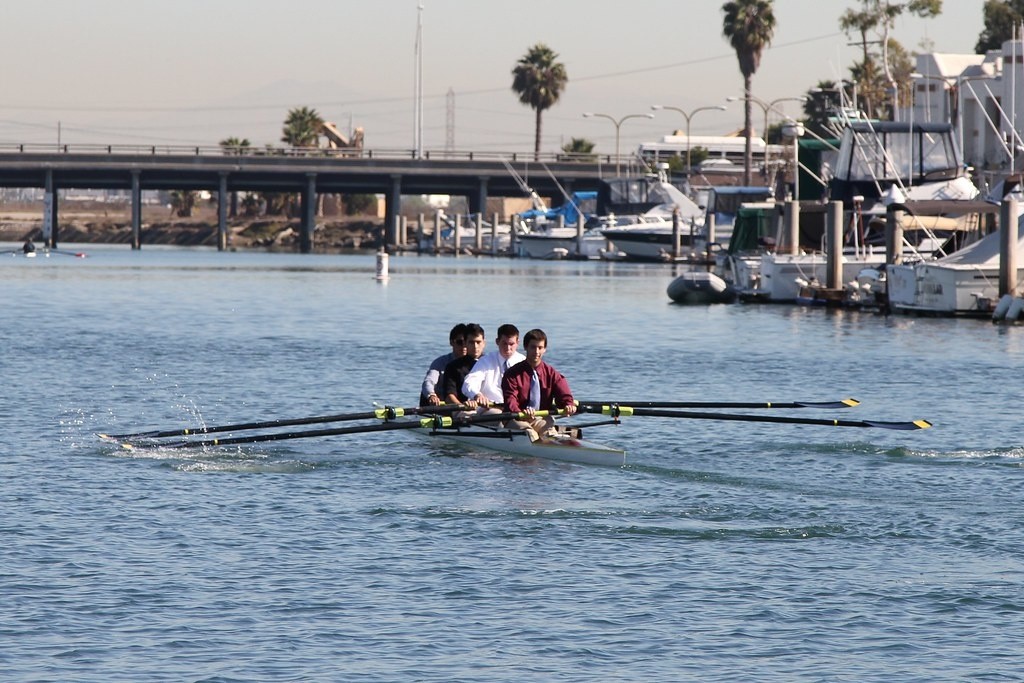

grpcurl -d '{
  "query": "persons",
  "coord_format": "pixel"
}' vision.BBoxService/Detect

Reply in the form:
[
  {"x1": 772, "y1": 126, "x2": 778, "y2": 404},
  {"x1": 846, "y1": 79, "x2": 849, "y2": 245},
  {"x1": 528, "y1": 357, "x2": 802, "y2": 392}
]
[
  {"x1": 23, "y1": 237, "x2": 36, "y2": 254},
  {"x1": 462, "y1": 323, "x2": 525, "y2": 423},
  {"x1": 419, "y1": 323, "x2": 468, "y2": 408},
  {"x1": 500, "y1": 328, "x2": 578, "y2": 445},
  {"x1": 441, "y1": 324, "x2": 487, "y2": 417}
]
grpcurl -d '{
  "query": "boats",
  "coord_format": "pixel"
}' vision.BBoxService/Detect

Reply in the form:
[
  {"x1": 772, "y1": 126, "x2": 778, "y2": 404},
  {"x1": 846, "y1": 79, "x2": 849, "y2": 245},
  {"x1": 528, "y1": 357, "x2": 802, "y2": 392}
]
[
  {"x1": 711, "y1": 18, "x2": 1024, "y2": 325},
  {"x1": 373, "y1": 402, "x2": 626, "y2": 466},
  {"x1": 418, "y1": 139, "x2": 729, "y2": 263}
]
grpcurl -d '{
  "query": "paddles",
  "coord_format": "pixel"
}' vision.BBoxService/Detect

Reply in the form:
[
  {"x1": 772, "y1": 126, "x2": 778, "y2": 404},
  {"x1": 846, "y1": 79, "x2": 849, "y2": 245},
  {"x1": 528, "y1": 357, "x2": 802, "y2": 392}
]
[
  {"x1": 574, "y1": 406, "x2": 933, "y2": 431},
  {"x1": 93, "y1": 401, "x2": 531, "y2": 452},
  {"x1": 551, "y1": 398, "x2": 861, "y2": 409}
]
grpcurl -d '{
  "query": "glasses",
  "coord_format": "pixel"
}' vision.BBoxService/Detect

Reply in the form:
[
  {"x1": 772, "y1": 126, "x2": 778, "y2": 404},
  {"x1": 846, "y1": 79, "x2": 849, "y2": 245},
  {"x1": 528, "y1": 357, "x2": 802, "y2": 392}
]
[{"x1": 453, "y1": 339, "x2": 466, "y2": 346}]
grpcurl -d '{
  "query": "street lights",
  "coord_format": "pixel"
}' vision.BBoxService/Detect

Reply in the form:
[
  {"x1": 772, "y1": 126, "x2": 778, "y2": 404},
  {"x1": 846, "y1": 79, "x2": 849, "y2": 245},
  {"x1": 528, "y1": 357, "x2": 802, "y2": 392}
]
[
  {"x1": 582, "y1": 112, "x2": 653, "y2": 178},
  {"x1": 651, "y1": 105, "x2": 727, "y2": 182}
]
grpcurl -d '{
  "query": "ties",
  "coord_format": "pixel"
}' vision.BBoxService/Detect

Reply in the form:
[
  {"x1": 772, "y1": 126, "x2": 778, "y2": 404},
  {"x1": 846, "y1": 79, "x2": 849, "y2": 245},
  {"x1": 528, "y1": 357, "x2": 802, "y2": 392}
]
[
  {"x1": 527, "y1": 370, "x2": 540, "y2": 411},
  {"x1": 504, "y1": 360, "x2": 510, "y2": 372}
]
